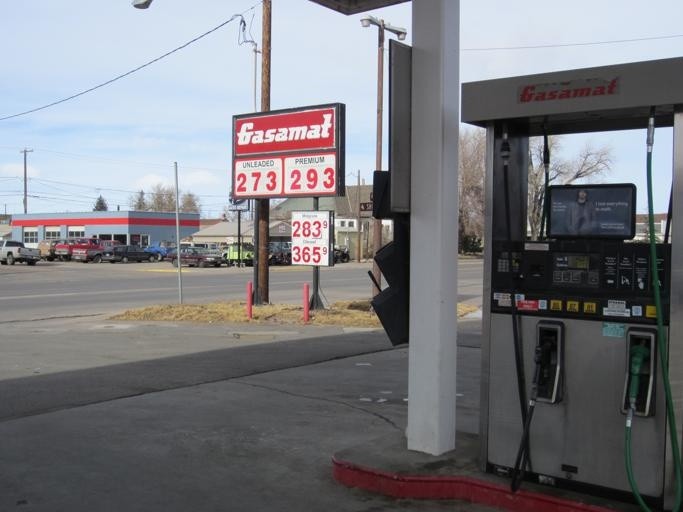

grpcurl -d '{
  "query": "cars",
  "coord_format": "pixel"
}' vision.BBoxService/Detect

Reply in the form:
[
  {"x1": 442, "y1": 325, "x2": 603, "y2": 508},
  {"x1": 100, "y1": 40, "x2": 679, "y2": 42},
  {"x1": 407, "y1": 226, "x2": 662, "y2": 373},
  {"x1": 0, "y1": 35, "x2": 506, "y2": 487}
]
[{"x1": 113, "y1": 241, "x2": 291, "y2": 268}]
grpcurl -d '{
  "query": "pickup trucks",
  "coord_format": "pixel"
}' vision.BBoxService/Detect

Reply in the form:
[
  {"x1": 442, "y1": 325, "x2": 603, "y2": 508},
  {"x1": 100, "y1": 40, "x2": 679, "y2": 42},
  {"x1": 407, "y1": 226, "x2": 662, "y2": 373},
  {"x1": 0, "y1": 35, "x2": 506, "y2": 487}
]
[{"x1": 0, "y1": 240, "x2": 42, "y2": 265}]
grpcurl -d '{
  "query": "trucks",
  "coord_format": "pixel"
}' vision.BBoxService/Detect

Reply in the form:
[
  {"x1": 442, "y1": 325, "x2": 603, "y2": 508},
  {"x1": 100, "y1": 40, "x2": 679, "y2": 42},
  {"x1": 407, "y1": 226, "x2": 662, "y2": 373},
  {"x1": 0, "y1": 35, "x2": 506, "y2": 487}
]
[{"x1": 37, "y1": 237, "x2": 124, "y2": 264}]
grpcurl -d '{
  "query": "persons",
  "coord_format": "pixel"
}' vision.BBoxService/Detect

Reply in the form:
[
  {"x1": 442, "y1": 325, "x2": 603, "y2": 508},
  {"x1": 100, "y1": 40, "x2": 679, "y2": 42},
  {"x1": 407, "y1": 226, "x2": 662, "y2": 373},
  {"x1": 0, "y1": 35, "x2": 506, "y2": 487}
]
[{"x1": 565, "y1": 189, "x2": 597, "y2": 235}]
